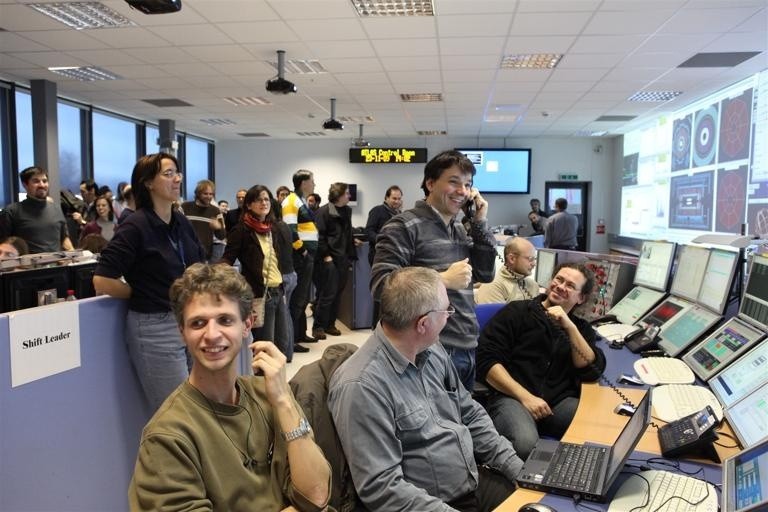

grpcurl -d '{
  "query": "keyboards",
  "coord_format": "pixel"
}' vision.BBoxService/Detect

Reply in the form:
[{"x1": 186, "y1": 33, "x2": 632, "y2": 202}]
[
  {"x1": 633, "y1": 356, "x2": 696, "y2": 386},
  {"x1": 653, "y1": 385, "x2": 724, "y2": 426},
  {"x1": 607, "y1": 470, "x2": 719, "y2": 512},
  {"x1": 596, "y1": 324, "x2": 640, "y2": 343}
]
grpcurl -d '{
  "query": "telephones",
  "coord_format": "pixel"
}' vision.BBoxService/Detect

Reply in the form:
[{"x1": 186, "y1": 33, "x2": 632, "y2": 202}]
[
  {"x1": 466, "y1": 199, "x2": 476, "y2": 219},
  {"x1": 590, "y1": 314, "x2": 620, "y2": 327},
  {"x1": 623, "y1": 323, "x2": 661, "y2": 354}
]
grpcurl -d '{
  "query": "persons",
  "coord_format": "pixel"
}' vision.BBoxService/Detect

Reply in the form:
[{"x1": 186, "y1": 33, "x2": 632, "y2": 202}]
[
  {"x1": 328, "y1": 270, "x2": 529, "y2": 511},
  {"x1": 477, "y1": 260, "x2": 607, "y2": 456},
  {"x1": 127, "y1": 262, "x2": 336, "y2": 511}
]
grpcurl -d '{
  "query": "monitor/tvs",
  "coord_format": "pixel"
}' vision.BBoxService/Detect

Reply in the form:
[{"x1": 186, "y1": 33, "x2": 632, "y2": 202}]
[
  {"x1": 681, "y1": 317, "x2": 767, "y2": 383},
  {"x1": 721, "y1": 437, "x2": 768, "y2": 512},
  {"x1": 669, "y1": 246, "x2": 712, "y2": 304},
  {"x1": 723, "y1": 383, "x2": 768, "y2": 450},
  {"x1": 696, "y1": 248, "x2": 741, "y2": 316},
  {"x1": 736, "y1": 255, "x2": 768, "y2": 334},
  {"x1": 454, "y1": 147, "x2": 532, "y2": 195},
  {"x1": 636, "y1": 295, "x2": 694, "y2": 338},
  {"x1": 632, "y1": 240, "x2": 677, "y2": 293},
  {"x1": 657, "y1": 306, "x2": 724, "y2": 359},
  {"x1": 606, "y1": 286, "x2": 667, "y2": 326},
  {"x1": 707, "y1": 339, "x2": 768, "y2": 410}
]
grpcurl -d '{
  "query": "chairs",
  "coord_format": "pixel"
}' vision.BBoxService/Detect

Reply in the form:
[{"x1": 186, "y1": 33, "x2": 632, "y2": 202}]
[{"x1": 474, "y1": 300, "x2": 510, "y2": 333}]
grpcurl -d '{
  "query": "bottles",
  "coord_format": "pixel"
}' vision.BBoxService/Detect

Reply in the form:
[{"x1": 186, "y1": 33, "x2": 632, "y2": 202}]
[{"x1": 65, "y1": 289, "x2": 78, "y2": 301}]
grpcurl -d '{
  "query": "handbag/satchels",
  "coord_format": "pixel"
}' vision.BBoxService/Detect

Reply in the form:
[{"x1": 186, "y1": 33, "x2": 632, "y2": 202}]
[{"x1": 238, "y1": 297, "x2": 266, "y2": 330}]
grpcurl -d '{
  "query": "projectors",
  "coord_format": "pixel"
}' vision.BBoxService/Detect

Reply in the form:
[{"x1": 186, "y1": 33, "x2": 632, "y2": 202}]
[
  {"x1": 324, "y1": 120, "x2": 341, "y2": 129},
  {"x1": 267, "y1": 79, "x2": 296, "y2": 95},
  {"x1": 353, "y1": 138, "x2": 370, "y2": 148},
  {"x1": 122, "y1": 0, "x2": 182, "y2": 14}
]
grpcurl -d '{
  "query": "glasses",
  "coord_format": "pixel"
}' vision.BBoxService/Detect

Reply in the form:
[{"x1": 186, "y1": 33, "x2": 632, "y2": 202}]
[
  {"x1": 518, "y1": 254, "x2": 537, "y2": 262},
  {"x1": 423, "y1": 305, "x2": 455, "y2": 317},
  {"x1": 160, "y1": 171, "x2": 183, "y2": 180}
]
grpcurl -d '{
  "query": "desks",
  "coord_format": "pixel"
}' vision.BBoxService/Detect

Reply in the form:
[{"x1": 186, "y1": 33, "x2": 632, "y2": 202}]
[{"x1": 489, "y1": 314, "x2": 744, "y2": 512}]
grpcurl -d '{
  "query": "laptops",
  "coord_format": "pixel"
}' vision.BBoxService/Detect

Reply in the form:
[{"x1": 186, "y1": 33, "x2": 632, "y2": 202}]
[{"x1": 515, "y1": 386, "x2": 653, "y2": 504}]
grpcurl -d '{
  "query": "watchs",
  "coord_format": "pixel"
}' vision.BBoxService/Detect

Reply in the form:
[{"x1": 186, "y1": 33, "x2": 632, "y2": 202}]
[{"x1": 282, "y1": 416, "x2": 312, "y2": 440}]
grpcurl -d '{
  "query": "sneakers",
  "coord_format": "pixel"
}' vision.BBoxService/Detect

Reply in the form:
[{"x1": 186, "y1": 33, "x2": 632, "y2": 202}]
[
  {"x1": 312, "y1": 328, "x2": 326, "y2": 340},
  {"x1": 294, "y1": 334, "x2": 318, "y2": 343},
  {"x1": 323, "y1": 325, "x2": 342, "y2": 336},
  {"x1": 287, "y1": 343, "x2": 310, "y2": 353}
]
